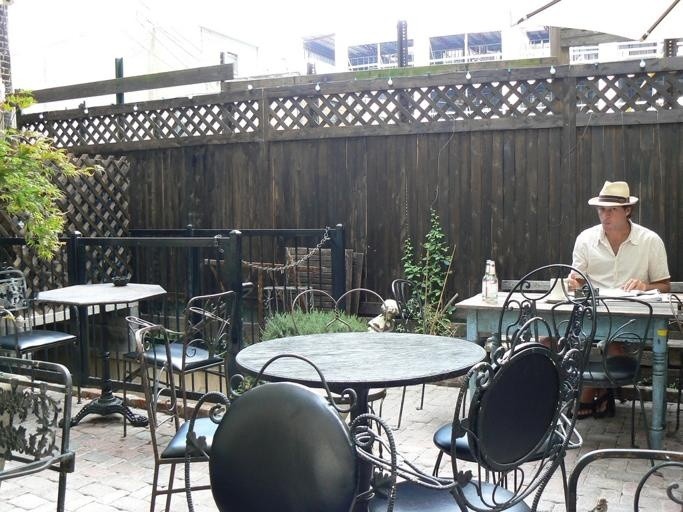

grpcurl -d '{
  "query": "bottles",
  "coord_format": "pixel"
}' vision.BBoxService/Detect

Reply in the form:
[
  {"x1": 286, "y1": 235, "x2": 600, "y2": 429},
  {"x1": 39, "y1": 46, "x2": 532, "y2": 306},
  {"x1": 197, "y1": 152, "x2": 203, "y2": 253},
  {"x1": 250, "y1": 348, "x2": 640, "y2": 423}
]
[
  {"x1": 486, "y1": 261, "x2": 498, "y2": 303},
  {"x1": 482, "y1": 260, "x2": 491, "y2": 301}
]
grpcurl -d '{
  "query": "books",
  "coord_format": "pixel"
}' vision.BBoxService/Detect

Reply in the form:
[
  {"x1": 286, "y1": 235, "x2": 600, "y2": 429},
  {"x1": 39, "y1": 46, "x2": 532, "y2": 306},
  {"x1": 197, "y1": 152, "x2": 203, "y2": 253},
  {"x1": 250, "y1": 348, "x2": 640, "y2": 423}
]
[{"x1": 597, "y1": 287, "x2": 656, "y2": 297}]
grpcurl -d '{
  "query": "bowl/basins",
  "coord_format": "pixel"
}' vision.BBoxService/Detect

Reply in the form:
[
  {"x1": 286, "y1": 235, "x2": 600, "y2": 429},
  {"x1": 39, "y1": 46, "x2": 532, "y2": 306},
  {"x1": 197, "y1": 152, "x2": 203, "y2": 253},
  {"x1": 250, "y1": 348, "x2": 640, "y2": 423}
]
[{"x1": 112, "y1": 276, "x2": 129, "y2": 286}]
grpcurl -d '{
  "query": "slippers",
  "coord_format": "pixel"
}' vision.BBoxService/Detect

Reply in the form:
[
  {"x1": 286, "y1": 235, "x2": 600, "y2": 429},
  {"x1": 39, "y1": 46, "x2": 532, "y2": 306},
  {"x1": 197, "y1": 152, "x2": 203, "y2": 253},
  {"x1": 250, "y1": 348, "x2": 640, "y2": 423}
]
[
  {"x1": 576, "y1": 402, "x2": 593, "y2": 419},
  {"x1": 593, "y1": 393, "x2": 609, "y2": 417}
]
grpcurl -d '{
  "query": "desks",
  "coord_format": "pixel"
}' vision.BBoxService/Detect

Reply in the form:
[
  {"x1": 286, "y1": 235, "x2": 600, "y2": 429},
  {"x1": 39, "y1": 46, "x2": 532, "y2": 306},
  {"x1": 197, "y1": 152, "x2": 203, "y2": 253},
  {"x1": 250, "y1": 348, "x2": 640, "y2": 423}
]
[
  {"x1": 236, "y1": 331, "x2": 488, "y2": 512},
  {"x1": 37, "y1": 282, "x2": 167, "y2": 428},
  {"x1": 455, "y1": 292, "x2": 678, "y2": 451}
]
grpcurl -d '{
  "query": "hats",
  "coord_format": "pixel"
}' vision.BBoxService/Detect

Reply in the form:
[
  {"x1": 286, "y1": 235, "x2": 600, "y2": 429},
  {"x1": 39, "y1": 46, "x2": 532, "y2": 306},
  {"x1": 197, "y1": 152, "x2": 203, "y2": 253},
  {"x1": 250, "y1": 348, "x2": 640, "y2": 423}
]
[{"x1": 588, "y1": 179, "x2": 639, "y2": 207}]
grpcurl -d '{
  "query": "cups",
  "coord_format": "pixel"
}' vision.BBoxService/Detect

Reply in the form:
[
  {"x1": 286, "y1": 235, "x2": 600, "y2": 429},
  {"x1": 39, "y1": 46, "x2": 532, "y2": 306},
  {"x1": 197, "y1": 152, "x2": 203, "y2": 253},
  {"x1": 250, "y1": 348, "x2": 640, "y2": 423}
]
[
  {"x1": 550, "y1": 278, "x2": 569, "y2": 301},
  {"x1": 576, "y1": 271, "x2": 587, "y2": 289}
]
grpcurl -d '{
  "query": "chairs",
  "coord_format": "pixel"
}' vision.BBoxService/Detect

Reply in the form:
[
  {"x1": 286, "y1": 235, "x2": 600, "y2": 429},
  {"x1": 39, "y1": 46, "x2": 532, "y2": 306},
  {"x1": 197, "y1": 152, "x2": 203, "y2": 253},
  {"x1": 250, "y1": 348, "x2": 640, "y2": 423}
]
[
  {"x1": 185, "y1": 354, "x2": 397, "y2": 512},
  {"x1": 551, "y1": 296, "x2": 653, "y2": 450},
  {"x1": 367, "y1": 340, "x2": 585, "y2": 512},
  {"x1": 432, "y1": 264, "x2": 597, "y2": 512},
  {"x1": 123, "y1": 291, "x2": 237, "y2": 437},
  {"x1": 135, "y1": 324, "x2": 224, "y2": 512},
  {"x1": 0, "y1": 270, "x2": 81, "y2": 404},
  {"x1": 391, "y1": 279, "x2": 468, "y2": 429},
  {"x1": 291, "y1": 288, "x2": 388, "y2": 460}
]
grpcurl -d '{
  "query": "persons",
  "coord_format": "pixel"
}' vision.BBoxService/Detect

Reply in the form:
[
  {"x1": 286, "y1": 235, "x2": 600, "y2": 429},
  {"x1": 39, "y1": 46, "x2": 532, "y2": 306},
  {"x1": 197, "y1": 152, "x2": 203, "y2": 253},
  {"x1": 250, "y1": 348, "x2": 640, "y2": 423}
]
[{"x1": 539, "y1": 180, "x2": 671, "y2": 419}]
